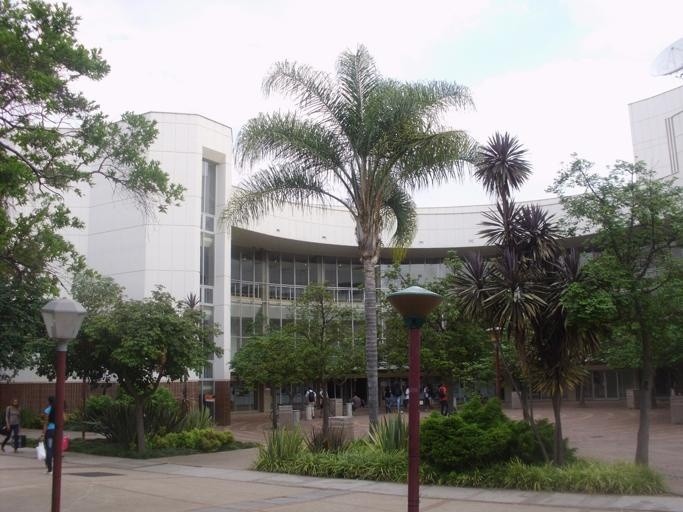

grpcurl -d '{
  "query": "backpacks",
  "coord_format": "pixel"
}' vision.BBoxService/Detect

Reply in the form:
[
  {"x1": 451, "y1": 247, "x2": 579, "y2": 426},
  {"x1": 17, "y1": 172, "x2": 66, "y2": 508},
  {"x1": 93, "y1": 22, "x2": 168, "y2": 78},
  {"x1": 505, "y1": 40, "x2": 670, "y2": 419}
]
[
  {"x1": 384, "y1": 391, "x2": 391, "y2": 396},
  {"x1": 307, "y1": 390, "x2": 314, "y2": 402}
]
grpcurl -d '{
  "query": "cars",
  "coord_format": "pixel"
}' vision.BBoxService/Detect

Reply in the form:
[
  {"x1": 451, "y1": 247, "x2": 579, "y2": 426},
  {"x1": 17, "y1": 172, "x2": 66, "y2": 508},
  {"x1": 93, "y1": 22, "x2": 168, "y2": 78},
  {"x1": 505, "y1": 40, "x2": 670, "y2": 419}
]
[{"x1": 403, "y1": 392, "x2": 432, "y2": 412}]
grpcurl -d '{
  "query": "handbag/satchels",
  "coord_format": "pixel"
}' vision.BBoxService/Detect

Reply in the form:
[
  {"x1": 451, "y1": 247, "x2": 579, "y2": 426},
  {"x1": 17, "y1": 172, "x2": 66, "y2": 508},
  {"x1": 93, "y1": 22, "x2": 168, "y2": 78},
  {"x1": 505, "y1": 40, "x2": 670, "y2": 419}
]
[
  {"x1": 1, "y1": 426, "x2": 9, "y2": 436},
  {"x1": 439, "y1": 390, "x2": 449, "y2": 397},
  {"x1": 62, "y1": 434, "x2": 69, "y2": 451}
]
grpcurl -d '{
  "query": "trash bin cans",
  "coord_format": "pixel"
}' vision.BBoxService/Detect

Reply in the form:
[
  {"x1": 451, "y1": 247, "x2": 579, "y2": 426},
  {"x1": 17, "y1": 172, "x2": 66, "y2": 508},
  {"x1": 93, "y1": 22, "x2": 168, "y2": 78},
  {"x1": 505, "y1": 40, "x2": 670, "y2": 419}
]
[
  {"x1": 294, "y1": 410, "x2": 300, "y2": 421},
  {"x1": 326, "y1": 416, "x2": 354, "y2": 447},
  {"x1": 669, "y1": 395, "x2": 683, "y2": 426},
  {"x1": 625, "y1": 391, "x2": 641, "y2": 410},
  {"x1": 274, "y1": 405, "x2": 292, "y2": 427},
  {"x1": 511, "y1": 392, "x2": 521, "y2": 409},
  {"x1": 328, "y1": 398, "x2": 343, "y2": 417},
  {"x1": 344, "y1": 403, "x2": 353, "y2": 417},
  {"x1": 305, "y1": 405, "x2": 314, "y2": 419}
]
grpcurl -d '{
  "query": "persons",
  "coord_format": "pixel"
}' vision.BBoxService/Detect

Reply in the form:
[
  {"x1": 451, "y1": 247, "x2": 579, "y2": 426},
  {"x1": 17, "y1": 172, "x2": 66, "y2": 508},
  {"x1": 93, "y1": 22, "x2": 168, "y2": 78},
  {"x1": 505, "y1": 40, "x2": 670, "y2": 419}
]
[
  {"x1": 40, "y1": 395, "x2": 67, "y2": 474},
  {"x1": 299, "y1": 381, "x2": 450, "y2": 416},
  {"x1": 2, "y1": 397, "x2": 22, "y2": 454}
]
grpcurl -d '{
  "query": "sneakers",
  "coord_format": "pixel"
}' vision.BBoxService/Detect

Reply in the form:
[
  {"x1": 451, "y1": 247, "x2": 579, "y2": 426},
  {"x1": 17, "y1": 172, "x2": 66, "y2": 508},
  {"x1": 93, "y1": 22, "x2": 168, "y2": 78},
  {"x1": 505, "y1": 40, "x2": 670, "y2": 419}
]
[
  {"x1": 1, "y1": 446, "x2": 20, "y2": 453},
  {"x1": 42, "y1": 463, "x2": 53, "y2": 476}
]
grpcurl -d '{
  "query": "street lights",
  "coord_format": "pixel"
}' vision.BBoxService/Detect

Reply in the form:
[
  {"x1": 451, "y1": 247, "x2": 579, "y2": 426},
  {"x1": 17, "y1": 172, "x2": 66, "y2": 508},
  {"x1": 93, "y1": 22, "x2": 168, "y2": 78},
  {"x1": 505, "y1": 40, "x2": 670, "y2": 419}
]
[
  {"x1": 486, "y1": 327, "x2": 507, "y2": 398},
  {"x1": 387, "y1": 283, "x2": 442, "y2": 511},
  {"x1": 38, "y1": 296, "x2": 88, "y2": 510}
]
